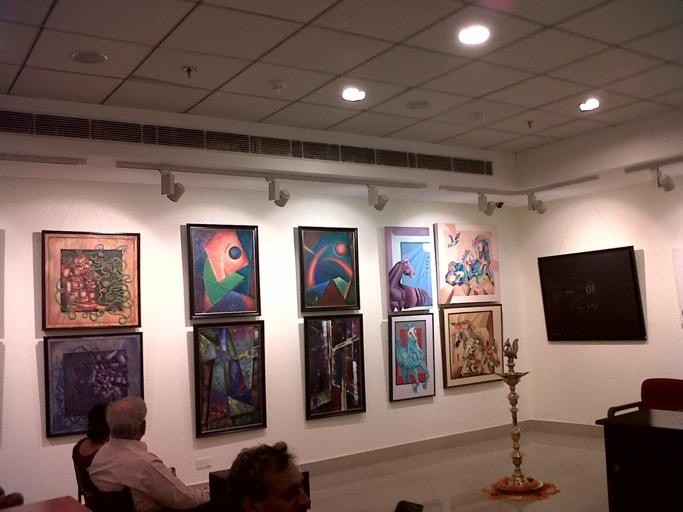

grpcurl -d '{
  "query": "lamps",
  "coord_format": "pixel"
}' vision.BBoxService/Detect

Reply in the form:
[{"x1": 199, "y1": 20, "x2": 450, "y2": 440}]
[
  {"x1": 115, "y1": 161, "x2": 426, "y2": 211},
  {"x1": 625, "y1": 155, "x2": 683, "y2": 192},
  {"x1": 439, "y1": 176, "x2": 599, "y2": 216}
]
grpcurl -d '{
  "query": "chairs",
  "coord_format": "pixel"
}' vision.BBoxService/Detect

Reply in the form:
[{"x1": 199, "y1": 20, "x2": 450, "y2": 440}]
[
  {"x1": 76, "y1": 462, "x2": 135, "y2": 512},
  {"x1": 608, "y1": 379, "x2": 683, "y2": 417}
]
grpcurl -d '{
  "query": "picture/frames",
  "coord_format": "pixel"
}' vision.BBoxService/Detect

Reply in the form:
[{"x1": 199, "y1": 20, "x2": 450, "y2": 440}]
[
  {"x1": 193, "y1": 321, "x2": 266, "y2": 438},
  {"x1": 298, "y1": 226, "x2": 360, "y2": 312},
  {"x1": 42, "y1": 230, "x2": 141, "y2": 331},
  {"x1": 187, "y1": 224, "x2": 261, "y2": 319},
  {"x1": 385, "y1": 223, "x2": 504, "y2": 402},
  {"x1": 304, "y1": 314, "x2": 366, "y2": 420},
  {"x1": 44, "y1": 331, "x2": 145, "y2": 438}
]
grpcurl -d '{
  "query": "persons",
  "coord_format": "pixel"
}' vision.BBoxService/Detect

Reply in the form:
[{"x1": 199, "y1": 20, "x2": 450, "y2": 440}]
[
  {"x1": 71, "y1": 401, "x2": 111, "y2": 498},
  {"x1": 227, "y1": 439, "x2": 312, "y2": 512},
  {"x1": 86, "y1": 395, "x2": 211, "y2": 512}
]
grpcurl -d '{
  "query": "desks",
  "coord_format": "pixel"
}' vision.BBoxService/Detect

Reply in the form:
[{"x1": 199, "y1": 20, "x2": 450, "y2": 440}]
[{"x1": 596, "y1": 409, "x2": 683, "y2": 512}]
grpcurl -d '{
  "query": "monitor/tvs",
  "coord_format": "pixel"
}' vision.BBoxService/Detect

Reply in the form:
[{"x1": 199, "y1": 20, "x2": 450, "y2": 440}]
[{"x1": 538, "y1": 246, "x2": 647, "y2": 341}]
